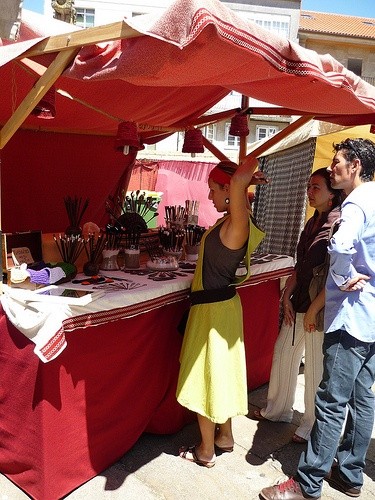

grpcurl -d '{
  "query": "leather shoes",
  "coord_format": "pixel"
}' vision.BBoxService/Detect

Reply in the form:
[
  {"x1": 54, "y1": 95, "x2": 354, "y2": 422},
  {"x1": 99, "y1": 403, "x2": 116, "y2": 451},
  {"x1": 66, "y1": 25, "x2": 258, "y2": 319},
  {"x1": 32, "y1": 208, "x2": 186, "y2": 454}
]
[
  {"x1": 258, "y1": 477, "x2": 321, "y2": 500},
  {"x1": 324, "y1": 465, "x2": 360, "y2": 496}
]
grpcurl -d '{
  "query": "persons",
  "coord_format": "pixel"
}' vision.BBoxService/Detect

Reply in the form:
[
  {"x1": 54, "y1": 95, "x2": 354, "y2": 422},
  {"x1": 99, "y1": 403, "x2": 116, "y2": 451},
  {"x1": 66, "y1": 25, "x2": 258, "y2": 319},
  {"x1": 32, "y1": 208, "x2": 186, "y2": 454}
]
[
  {"x1": 175, "y1": 155, "x2": 269, "y2": 469},
  {"x1": 252, "y1": 167, "x2": 347, "y2": 443},
  {"x1": 260, "y1": 138, "x2": 375, "y2": 500}
]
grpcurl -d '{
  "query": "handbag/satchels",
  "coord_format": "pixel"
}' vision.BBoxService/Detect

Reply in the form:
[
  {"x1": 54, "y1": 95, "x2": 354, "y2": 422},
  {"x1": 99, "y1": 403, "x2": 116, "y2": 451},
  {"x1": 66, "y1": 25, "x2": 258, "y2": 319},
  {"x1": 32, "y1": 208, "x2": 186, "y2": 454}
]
[{"x1": 309, "y1": 263, "x2": 328, "y2": 331}]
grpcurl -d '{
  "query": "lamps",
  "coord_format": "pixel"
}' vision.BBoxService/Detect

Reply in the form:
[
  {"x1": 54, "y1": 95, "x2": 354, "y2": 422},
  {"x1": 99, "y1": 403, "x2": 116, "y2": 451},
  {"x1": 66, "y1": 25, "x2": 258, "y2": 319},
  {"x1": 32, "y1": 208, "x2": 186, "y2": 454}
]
[
  {"x1": 229, "y1": 115, "x2": 249, "y2": 137},
  {"x1": 111, "y1": 121, "x2": 145, "y2": 156},
  {"x1": 31, "y1": 79, "x2": 56, "y2": 120},
  {"x1": 182, "y1": 129, "x2": 205, "y2": 156}
]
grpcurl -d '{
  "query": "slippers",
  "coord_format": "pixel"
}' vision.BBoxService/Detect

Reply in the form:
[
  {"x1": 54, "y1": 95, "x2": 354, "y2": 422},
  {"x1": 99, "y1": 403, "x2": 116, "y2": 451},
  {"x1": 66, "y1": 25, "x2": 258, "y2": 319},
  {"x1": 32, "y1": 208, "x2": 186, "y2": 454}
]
[
  {"x1": 179, "y1": 444, "x2": 216, "y2": 467},
  {"x1": 214, "y1": 440, "x2": 234, "y2": 453}
]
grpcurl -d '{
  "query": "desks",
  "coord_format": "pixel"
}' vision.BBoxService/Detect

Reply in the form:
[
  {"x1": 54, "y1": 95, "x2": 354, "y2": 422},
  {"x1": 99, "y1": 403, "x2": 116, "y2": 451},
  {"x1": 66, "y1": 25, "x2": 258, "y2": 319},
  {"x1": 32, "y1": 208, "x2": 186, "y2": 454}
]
[{"x1": 0, "y1": 254, "x2": 294, "y2": 500}]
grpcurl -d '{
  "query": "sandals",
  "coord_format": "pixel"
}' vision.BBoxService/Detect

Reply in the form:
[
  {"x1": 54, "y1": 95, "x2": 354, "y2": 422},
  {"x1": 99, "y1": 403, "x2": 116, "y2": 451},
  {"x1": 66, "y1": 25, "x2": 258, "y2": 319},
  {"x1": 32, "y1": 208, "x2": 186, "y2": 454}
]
[
  {"x1": 293, "y1": 435, "x2": 307, "y2": 443},
  {"x1": 253, "y1": 408, "x2": 267, "y2": 419}
]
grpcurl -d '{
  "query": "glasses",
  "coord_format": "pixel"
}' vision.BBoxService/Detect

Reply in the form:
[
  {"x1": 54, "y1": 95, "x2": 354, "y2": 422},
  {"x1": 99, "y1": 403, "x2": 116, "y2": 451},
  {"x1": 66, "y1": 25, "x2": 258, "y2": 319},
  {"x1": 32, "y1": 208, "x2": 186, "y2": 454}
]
[{"x1": 345, "y1": 138, "x2": 362, "y2": 160}]
